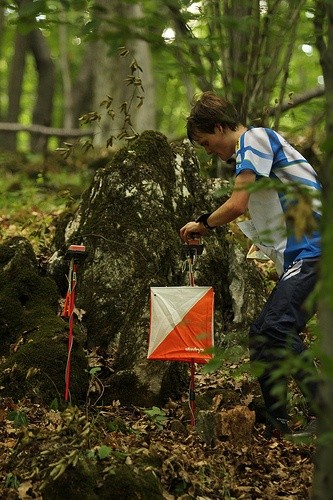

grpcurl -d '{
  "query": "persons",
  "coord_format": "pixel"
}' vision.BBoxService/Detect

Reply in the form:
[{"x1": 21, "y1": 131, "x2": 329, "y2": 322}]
[{"x1": 178, "y1": 92, "x2": 325, "y2": 437}]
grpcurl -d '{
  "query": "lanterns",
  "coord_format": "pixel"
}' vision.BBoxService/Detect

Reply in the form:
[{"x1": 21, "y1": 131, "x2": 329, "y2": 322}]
[{"x1": 149, "y1": 249, "x2": 222, "y2": 428}]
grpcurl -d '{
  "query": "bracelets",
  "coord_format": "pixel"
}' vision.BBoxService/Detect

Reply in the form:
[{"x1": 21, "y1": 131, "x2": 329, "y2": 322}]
[{"x1": 196, "y1": 212, "x2": 218, "y2": 231}]
